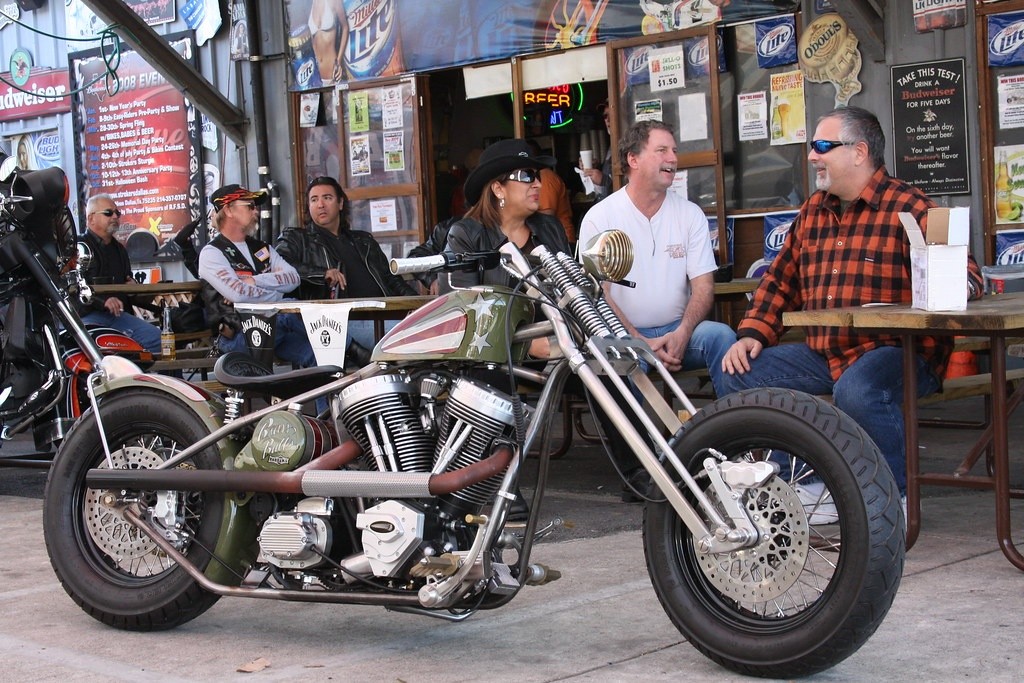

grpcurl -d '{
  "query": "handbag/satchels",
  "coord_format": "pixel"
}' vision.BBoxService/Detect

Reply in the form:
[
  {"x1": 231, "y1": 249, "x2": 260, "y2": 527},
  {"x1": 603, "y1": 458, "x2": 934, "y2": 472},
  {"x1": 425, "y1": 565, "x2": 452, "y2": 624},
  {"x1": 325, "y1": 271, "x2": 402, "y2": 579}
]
[{"x1": 157, "y1": 300, "x2": 207, "y2": 334}]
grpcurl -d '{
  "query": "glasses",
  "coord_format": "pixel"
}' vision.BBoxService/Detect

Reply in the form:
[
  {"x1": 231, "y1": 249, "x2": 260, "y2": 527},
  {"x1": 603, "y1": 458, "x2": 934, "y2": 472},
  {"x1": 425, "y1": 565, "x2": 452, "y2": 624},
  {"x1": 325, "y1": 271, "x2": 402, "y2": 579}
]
[
  {"x1": 810, "y1": 139, "x2": 855, "y2": 155},
  {"x1": 309, "y1": 176, "x2": 333, "y2": 184},
  {"x1": 94, "y1": 209, "x2": 121, "y2": 218},
  {"x1": 229, "y1": 201, "x2": 262, "y2": 211},
  {"x1": 601, "y1": 112, "x2": 609, "y2": 119},
  {"x1": 506, "y1": 167, "x2": 542, "y2": 183}
]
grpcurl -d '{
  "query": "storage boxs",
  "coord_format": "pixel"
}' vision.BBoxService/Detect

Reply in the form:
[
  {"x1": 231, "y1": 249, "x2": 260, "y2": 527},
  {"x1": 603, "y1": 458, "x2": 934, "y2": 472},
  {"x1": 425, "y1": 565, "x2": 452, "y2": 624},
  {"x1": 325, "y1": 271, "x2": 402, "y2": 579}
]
[{"x1": 897, "y1": 204, "x2": 971, "y2": 311}]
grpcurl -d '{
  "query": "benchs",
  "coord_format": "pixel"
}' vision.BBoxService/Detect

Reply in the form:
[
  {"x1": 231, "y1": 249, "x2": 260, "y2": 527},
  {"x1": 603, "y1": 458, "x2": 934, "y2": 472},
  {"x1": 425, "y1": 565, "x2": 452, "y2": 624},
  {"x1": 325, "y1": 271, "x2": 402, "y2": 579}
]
[
  {"x1": 899, "y1": 335, "x2": 1023, "y2": 476},
  {"x1": 147, "y1": 345, "x2": 261, "y2": 418}
]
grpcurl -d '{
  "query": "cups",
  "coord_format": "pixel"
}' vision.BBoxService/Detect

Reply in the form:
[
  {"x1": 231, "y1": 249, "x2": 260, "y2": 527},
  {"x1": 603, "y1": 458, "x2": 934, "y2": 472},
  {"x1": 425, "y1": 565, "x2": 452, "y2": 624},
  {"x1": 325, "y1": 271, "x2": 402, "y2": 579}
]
[
  {"x1": 579, "y1": 130, "x2": 606, "y2": 170},
  {"x1": 778, "y1": 100, "x2": 791, "y2": 139}
]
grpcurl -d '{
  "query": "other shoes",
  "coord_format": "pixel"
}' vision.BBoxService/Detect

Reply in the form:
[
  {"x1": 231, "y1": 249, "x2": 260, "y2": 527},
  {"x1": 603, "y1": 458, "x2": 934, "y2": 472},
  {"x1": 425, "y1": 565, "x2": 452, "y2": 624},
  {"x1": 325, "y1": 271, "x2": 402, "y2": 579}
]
[
  {"x1": 775, "y1": 480, "x2": 839, "y2": 525},
  {"x1": 621, "y1": 466, "x2": 649, "y2": 502},
  {"x1": 345, "y1": 338, "x2": 372, "y2": 368}
]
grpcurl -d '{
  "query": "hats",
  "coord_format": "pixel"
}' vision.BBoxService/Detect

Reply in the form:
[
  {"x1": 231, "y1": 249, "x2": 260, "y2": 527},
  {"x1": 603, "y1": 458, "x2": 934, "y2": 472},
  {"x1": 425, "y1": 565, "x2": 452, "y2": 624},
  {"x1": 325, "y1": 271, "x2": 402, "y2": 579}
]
[
  {"x1": 596, "y1": 98, "x2": 609, "y2": 114},
  {"x1": 463, "y1": 138, "x2": 558, "y2": 207},
  {"x1": 210, "y1": 184, "x2": 268, "y2": 214}
]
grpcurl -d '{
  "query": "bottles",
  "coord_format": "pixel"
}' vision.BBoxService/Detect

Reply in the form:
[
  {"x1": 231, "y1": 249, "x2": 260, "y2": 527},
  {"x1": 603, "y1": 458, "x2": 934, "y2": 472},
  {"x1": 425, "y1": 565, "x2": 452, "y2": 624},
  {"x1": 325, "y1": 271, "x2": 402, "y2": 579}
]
[
  {"x1": 772, "y1": 96, "x2": 782, "y2": 139},
  {"x1": 996, "y1": 161, "x2": 1011, "y2": 219},
  {"x1": 161, "y1": 307, "x2": 176, "y2": 361}
]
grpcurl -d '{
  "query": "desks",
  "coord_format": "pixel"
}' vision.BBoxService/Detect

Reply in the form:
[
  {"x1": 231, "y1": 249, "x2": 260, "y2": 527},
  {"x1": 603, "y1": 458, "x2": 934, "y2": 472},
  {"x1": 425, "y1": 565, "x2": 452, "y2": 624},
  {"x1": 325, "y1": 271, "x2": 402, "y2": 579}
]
[
  {"x1": 234, "y1": 295, "x2": 437, "y2": 425},
  {"x1": 89, "y1": 280, "x2": 212, "y2": 382},
  {"x1": 783, "y1": 291, "x2": 1024, "y2": 571}
]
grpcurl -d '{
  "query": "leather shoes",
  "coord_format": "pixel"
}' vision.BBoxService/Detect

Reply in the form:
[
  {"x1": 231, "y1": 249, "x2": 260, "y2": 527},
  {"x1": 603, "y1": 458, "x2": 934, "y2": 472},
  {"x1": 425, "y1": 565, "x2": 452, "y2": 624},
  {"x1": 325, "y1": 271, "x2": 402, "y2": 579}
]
[{"x1": 505, "y1": 480, "x2": 529, "y2": 521}]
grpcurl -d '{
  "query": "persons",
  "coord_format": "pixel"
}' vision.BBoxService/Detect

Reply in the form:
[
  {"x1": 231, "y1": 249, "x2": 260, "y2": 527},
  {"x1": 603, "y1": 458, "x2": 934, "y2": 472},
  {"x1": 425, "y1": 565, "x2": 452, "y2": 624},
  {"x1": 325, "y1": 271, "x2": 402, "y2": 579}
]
[
  {"x1": 16, "y1": 134, "x2": 38, "y2": 170},
  {"x1": 578, "y1": 95, "x2": 613, "y2": 202},
  {"x1": 272, "y1": 176, "x2": 422, "y2": 350},
  {"x1": 308, "y1": 0, "x2": 349, "y2": 86},
  {"x1": 434, "y1": 135, "x2": 572, "y2": 521},
  {"x1": 70, "y1": 193, "x2": 185, "y2": 378},
  {"x1": 718, "y1": 107, "x2": 983, "y2": 531},
  {"x1": 405, "y1": 211, "x2": 463, "y2": 296},
  {"x1": 577, "y1": 118, "x2": 740, "y2": 399},
  {"x1": 197, "y1": 183, "x2": 331, "y2": 416},
  {"x1": 528, "y1": 139, "x2": 576, "y2": 256}
]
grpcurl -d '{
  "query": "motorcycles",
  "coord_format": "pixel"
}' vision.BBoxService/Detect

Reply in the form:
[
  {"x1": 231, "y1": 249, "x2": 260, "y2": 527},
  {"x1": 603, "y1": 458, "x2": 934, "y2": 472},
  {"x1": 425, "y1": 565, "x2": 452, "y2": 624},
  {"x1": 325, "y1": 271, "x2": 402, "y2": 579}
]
[
  {"x1": 40, "y1": 224, "x2": 909, "y2": 681},
  {"x1": 0, "y1": 152, "x2": 158, "y2": 468}
]
